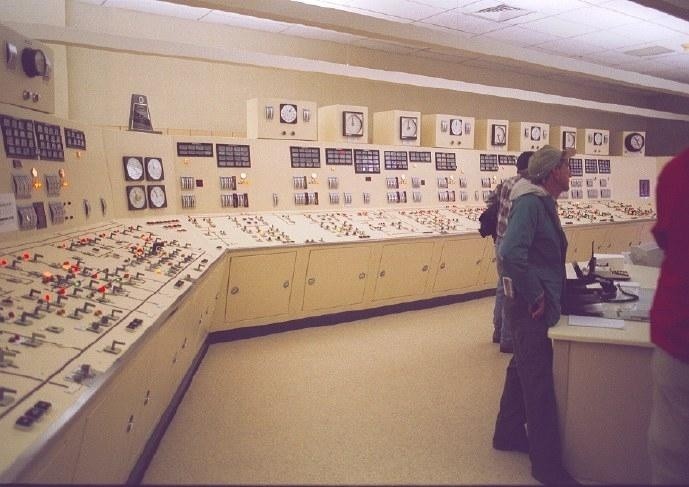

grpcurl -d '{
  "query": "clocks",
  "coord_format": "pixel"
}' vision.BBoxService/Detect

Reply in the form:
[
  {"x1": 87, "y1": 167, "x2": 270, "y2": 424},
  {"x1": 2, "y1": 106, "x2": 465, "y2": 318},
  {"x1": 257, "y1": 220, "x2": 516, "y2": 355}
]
[
  {"x1": 531, "y1": 126, "x2": 540, "y2": 141},
  {"x1": 492, "y1": 124, "x2": 506, "y2": 145},
  {"x1": 594, "y1": 133, "x2": 602, "y2": 145},
  {"x1": 280, "y1": 104, "x2": 297, "y2": 123},
  {"x1": 563, "y1": 131, "x2": 575, "y2": 150},
  {"x1": 625, "y1": 133, "x2": 644, "y2": 152},
  {"x1": 22, "y1": 48, "x2": 46, "y2": 77},
  {"x1": 123, "y1": 156, "x2": 167, "y2": 210},
  {"x1": 450, "y1": 119, "x2": 462, "y2": 135},
  {"x1": 400, "y1": 117, "x2": 417, "y2": 140},
  {"x1": 344, "y1": 111, "x2": 363, "y2": 135}
]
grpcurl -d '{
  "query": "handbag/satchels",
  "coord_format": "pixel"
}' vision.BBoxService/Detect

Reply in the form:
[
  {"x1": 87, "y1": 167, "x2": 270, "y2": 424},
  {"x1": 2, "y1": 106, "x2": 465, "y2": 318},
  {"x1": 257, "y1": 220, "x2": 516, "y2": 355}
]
[{"x1": 478, "y1": 201, "x2": 497, "y2": 238}]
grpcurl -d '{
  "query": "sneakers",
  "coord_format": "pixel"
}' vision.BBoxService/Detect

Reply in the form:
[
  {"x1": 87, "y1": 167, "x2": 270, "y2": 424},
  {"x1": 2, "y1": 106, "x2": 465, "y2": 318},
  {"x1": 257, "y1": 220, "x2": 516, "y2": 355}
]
[
  {"x1": 490, "y1": 333, "x2": 516, "y2": 355},
  {"x1": 491, "y1": 433, "x2": 535, "y2": 454},
  {"x1": 530, "y1": 464, "x2": 584, "y2": 487}
]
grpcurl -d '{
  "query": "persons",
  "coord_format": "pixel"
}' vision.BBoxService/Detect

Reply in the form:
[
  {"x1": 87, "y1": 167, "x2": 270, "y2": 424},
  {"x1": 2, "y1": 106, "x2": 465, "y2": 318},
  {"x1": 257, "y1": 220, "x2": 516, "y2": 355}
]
[
  {"x1": 484, "y1": 150, "x2": 536, "y2": 353},
  {"x1": 489, "y1": 141, "x2": 589, "y2": 486},
  {"x1": 643, "y1": 147, "x2": 688, "y2": 486}
]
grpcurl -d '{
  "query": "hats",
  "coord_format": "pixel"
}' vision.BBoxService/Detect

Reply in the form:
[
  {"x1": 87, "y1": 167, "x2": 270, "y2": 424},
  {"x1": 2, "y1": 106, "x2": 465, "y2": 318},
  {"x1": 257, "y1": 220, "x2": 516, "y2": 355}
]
[
  {"x1": 527, "y1": 143, "x2": 575, "y2": 182},
  {"x1": 516, "y1": 151, "x2": 533, "y2": 170}
]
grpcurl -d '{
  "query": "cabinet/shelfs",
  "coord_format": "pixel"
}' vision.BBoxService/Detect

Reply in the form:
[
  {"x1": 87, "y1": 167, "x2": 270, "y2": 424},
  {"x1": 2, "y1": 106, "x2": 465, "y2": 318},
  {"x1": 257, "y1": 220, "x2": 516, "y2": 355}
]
[
  {"x1": 219, "y1": 220, "x2": 658, "y2": 331},
  {"x1": 1, "y1": 250, "x2": 229, "y2": 486}
]
